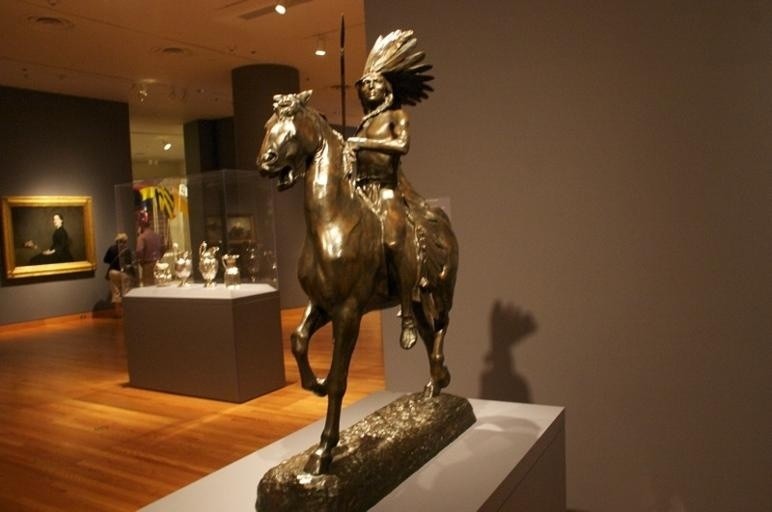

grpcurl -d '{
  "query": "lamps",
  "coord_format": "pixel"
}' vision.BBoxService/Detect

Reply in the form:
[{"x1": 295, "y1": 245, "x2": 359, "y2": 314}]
[
  {"x1": 274, "y1": 0, "x2": 288, "y2": 16},
  {"x1": 313, "y1": 33, "x2": 328, "y2": 56},
  {"x1": 160, "y1": 139, "x2": 171, "y2": 151}
]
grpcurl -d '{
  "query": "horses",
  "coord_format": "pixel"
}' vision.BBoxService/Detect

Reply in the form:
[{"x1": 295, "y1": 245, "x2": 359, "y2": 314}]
[{"x1": 254, "y1": 87, "x2": 460, "y2": 476}]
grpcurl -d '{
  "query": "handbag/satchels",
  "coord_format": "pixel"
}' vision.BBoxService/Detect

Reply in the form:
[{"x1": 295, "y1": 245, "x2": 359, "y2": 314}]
[{"x1": 105, "y1": 267, "x2": 110, "y2": 281}]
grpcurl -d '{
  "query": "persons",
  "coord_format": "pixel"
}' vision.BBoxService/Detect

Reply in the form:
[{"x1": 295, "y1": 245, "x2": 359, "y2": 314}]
[
  {"x1": 136, "y1": 220, "x2": 167, "y2": 284},
  {"x1": 350, "y1": 29, "x2": 436, "y2": 349},
  {"x1": 30, "y1": 214, "x2": 74, "y2": 265},
  {"x1": 104, "y1": 232, "x2": 138, "y2": 317}
]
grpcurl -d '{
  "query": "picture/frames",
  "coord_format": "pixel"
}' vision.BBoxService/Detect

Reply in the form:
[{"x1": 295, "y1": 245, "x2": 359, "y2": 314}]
[
  {"x1": 0, "y1": 194, "x2": 98, "y2": 282},
  {"x1": 206, "y1": 212, "x2": 254, "y2": 246}
]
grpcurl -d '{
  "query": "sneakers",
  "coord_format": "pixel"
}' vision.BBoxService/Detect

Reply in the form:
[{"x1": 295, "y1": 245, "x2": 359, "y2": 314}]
[{"x1": 113, "y1": 302, "x2": 124, "y2": 318}]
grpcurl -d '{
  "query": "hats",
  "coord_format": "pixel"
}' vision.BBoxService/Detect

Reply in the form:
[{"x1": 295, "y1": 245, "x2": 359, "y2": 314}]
[{"x1": 114, "y1": 232, "x2": 128, "y2": 241}]
